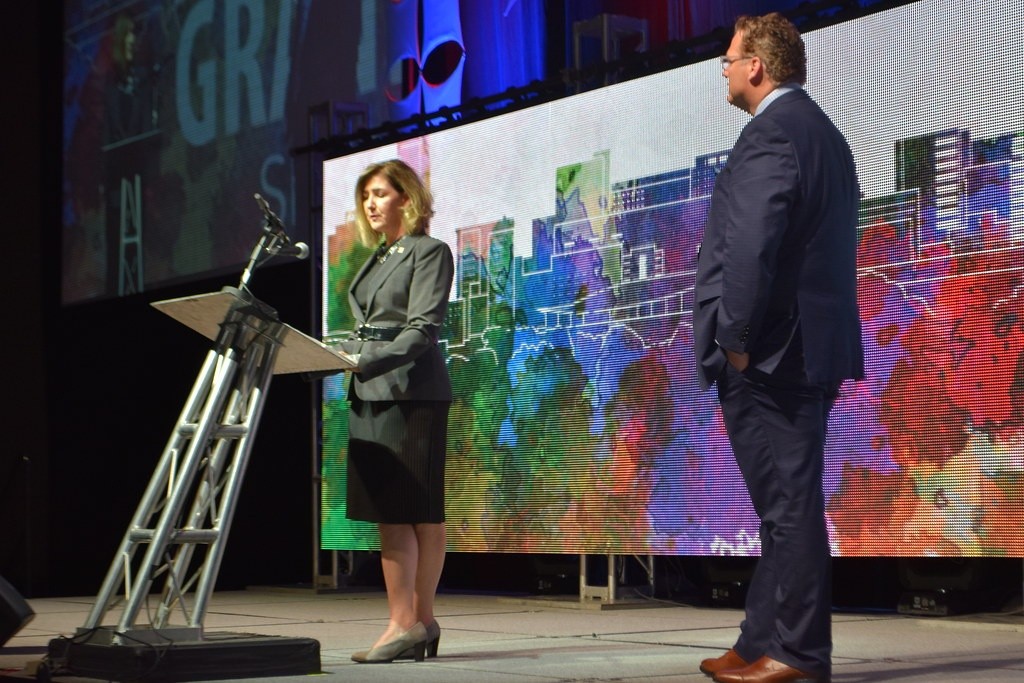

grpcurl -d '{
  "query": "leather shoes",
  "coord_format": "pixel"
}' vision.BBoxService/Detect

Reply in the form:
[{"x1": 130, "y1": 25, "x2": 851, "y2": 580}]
[
  {"x1": 700, "y1": 647, "x2": 751, "y2": 675},
  {"x1": 713, "y1": 654, "x2": 831, "y2": 683}
]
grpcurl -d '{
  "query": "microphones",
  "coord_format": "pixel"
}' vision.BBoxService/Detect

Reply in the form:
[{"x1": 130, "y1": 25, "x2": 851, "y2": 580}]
[{"x1": 268, "y1": 242, "x2": 309, "y2": 259}]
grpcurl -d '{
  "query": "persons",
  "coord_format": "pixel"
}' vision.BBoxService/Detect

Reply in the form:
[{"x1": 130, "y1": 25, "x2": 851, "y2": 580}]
[
  {"x1": 693, "y1": 13, "x2": 868, "y2": 683},
  {"x1": 338, "y1": 158, "x2": 454, "y2": 665}
]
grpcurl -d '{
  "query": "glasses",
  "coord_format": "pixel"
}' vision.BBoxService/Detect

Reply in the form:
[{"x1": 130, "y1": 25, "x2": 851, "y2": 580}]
[{"x1": 720, "y1": 55, "x2": 753, "y2": 70}]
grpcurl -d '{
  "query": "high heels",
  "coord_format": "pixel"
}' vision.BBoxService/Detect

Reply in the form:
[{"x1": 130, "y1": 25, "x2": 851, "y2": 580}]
[
  {"x1": 426, "y1": 619, "x2": 441, "y2": 657},
  {"x1": 351, "y1": 621, "x2": 427, "y2": 663}
]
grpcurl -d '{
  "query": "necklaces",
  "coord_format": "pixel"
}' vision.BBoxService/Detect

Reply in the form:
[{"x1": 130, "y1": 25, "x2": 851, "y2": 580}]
[{"x1": 375, "y1": 235, "x2": 408, "y2": 264}]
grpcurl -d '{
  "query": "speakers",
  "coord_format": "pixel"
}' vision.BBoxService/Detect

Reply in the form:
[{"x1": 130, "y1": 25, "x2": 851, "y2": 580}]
[{"x1": 0, "y1": 575, "x2": 35, "y2": 646}]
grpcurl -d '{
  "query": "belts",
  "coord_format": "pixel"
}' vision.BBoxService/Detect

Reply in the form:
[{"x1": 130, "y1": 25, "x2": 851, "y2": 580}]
[{"x1": 354, "y1": 324, "x2": 403, "y2": 341}]
